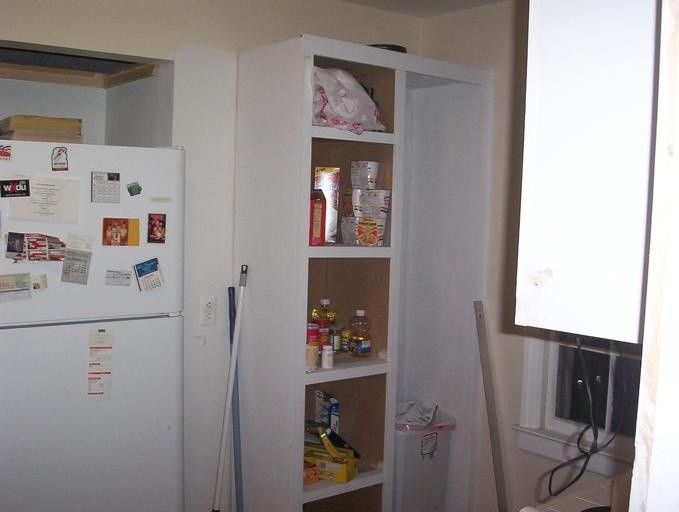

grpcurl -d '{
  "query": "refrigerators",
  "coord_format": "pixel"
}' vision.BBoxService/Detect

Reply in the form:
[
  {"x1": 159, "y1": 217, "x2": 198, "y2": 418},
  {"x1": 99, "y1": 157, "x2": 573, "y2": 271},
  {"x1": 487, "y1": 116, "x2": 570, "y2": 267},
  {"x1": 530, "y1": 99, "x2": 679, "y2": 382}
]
[{"x1": 0, "y1": 140, "x2": 186, "y2": 512}]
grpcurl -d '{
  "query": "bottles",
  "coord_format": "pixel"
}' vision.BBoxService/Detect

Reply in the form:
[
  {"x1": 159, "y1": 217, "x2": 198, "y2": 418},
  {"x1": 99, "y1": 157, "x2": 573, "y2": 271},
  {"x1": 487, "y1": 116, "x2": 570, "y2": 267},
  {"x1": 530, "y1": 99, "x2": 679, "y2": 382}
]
[
  {"x1": 328, "y1": 327, "x2": 341, "y2": 354},
  {"x1": 321, "y1": 345, "x2": 333, "y2": 369},
  {"x1": 312, "y1": 298, "x2": 338, "y2": 354},
  {"x1": 310, "y1": 189, "x2": 325, "y2": 245},
  {"x1": 340, "y1": 324, "x2": 352, "y2": 352},
  {"x1": 349, "y1": 310, "x2": 373, "y2": 358}
]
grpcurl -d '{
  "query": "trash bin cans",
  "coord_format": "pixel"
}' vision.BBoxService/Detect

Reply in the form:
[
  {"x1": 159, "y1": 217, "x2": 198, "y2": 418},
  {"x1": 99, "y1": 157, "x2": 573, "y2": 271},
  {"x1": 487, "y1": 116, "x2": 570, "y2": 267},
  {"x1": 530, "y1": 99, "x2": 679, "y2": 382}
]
[{"x1": 393, "y1": 401, "x2": 457, "y2": 512}]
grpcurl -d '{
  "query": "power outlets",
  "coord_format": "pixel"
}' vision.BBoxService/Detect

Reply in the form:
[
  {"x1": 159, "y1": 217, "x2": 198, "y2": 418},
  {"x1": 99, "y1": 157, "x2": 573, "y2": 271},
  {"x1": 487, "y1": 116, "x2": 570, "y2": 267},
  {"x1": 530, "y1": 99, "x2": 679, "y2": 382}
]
[{"x1": 198, "y1": 296, "x2": 215, "y2": 327}]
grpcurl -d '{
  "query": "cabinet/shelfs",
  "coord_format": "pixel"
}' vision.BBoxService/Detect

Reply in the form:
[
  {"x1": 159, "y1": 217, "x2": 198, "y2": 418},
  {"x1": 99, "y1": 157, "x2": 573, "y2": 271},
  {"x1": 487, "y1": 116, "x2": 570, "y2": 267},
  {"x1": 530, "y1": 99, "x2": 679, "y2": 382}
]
[{"x1": 229, "y1": 33, "x2": 494, "y2": 512}]
